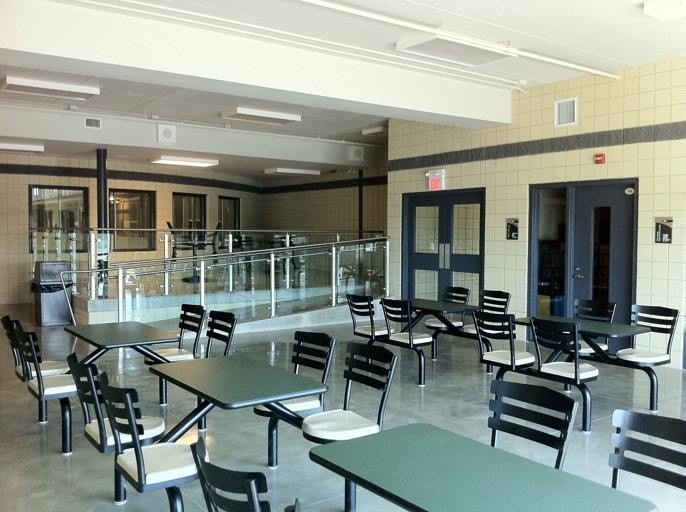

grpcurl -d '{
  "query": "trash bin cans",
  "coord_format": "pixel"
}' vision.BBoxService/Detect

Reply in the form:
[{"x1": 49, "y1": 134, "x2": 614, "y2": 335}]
[{"x1": 32, "y1": 260, "x2": 73, "y2": 326}]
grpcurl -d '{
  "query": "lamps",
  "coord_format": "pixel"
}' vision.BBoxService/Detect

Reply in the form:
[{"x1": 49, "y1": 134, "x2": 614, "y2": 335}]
[
  {"x1": 398, "y1": 26, "x2": 520, "y2": 69},
  {"x1": 361, "y1": 126, "x2": 387, "y2": 135},
  {"x1": 264, "y1": 166, "x2": 320, "y2": 178},
  {"x1": 218, "y1": 108, "x2": 301, "y2": 128},
  {"x1": 0, "y1": 75, "x2": 101, "y2": 102},
  {"x1": 146, "y1": 155, "x2": 220, "y2": 167},
  {"x1": 0, "y1": 142, "x2": 46, "y2": 156}
]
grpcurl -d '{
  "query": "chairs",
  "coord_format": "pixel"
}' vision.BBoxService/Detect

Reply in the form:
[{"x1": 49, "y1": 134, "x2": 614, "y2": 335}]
[
  {"x1": 609, "y1": 409, "x2": 685, "y2": 506},
  {"x1": 1, "y1": 304, "x2": 400, "y2": 512},
  {"x1": 487, "y1": 380, "x2": 580, "y2": 471},
  {"x1": 165, "y1": 219, "x2": 297, "y2": 284},
  {"x1": 347, "y1": 286, "x2": 684, "y2": 432}
]
